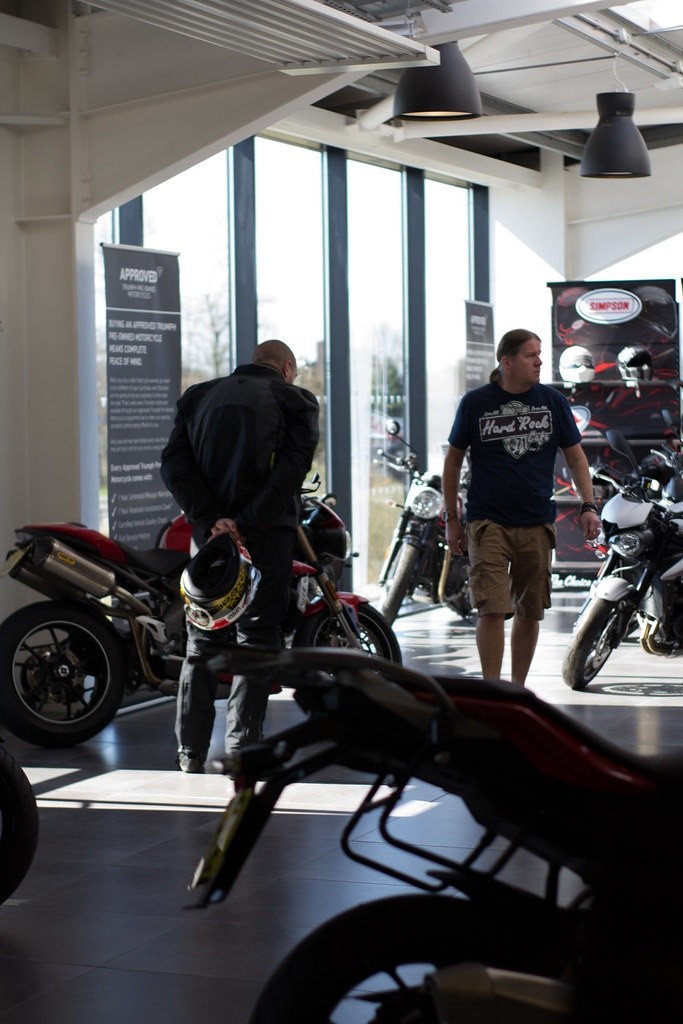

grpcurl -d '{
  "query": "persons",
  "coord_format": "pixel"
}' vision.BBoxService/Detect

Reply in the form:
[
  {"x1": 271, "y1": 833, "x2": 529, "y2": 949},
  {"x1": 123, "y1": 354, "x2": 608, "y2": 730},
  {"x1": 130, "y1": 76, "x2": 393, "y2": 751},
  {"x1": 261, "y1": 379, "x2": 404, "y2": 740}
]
[
  {"x1": 159, "y1": 339, "x2": 320, "y2": 775},
  {"x1": 442, "y1": 329, "x2": 602, "y2": 686}
]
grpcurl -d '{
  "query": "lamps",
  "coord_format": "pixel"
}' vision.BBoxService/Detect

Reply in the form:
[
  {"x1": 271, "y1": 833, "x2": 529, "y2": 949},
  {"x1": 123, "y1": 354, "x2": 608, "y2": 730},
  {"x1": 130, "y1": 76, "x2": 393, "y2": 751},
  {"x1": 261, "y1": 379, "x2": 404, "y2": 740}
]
[
  {"x1": 393, "y1": 40, "x2": 483, "y2": 122},
  {"x1": 581, "y1": 51, "x2": 651, "y2": 178}
]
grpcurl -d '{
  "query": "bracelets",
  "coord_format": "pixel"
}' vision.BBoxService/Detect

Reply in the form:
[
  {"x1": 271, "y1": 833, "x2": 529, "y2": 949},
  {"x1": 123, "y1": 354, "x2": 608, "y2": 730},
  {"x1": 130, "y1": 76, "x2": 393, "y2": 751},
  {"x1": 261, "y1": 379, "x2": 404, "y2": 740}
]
[{"x1": 580, "y1": 502, "x2": 598, "y2": 513}]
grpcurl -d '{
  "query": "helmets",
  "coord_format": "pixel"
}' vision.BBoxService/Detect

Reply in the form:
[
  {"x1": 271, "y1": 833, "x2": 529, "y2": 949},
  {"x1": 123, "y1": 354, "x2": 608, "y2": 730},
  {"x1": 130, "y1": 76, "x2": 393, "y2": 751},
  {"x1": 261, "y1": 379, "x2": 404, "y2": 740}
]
[
  {"x1": 559, "y1": 346, "x2": 595, "y2": 383},
  {"x1": 617, "y1": 346, "x2": 653, "y2": 381},
  {"x1": 179, "y1": 528, "x2": 261, "y2": 631}
]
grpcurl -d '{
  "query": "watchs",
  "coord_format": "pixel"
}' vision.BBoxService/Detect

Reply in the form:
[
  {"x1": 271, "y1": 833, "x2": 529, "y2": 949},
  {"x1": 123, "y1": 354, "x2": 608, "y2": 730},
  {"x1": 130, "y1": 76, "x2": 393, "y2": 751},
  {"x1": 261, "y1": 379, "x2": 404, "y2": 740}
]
[{"x1": 442, "y1": 510, "x2": 457, "y2": 522}]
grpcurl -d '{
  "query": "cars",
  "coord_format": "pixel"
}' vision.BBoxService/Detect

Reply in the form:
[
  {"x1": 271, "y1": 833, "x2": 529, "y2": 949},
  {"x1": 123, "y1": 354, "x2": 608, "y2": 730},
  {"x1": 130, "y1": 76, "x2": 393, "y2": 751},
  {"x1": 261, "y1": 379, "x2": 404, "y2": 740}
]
[{"x1": 311, "y1": 394, "x2": 407, "y2": 482}]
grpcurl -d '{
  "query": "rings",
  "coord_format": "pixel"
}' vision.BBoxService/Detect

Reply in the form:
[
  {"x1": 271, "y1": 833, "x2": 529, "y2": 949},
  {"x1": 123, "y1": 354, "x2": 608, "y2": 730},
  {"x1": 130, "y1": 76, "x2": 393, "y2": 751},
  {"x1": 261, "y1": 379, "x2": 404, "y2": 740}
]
[{"x1": 593, "y1": 531, "x2": 596, "y2": 534}]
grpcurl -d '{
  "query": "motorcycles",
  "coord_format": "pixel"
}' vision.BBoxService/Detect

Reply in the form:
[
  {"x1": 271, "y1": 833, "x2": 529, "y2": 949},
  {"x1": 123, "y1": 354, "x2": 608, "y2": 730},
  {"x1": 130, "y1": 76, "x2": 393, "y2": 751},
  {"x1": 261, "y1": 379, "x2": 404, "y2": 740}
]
[
  {"x1": 564, "y1": 428, "x2": 683, "y2": 692},
  {"x1": 0, "y1": 473, "x2": 403, "y2": 750},
  {"x1": 374, "y1": 420, "x2": 477, "y2": 631},
  {"x1": 178, "y1": 645, "x2": 683, "y2": 1024}
]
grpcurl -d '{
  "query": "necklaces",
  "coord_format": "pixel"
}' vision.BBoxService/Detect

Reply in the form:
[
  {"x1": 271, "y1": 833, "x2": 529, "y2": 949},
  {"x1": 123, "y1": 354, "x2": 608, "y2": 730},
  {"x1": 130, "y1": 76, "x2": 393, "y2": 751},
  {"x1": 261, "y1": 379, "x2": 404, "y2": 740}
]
[{"x1": 500, "y1": 390, "x2": 529, "y2": 423}]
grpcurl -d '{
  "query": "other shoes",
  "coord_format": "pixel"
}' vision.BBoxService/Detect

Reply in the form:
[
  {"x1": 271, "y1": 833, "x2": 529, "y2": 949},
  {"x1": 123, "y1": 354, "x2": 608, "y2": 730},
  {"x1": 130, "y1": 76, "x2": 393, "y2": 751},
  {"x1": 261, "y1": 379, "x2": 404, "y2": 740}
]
[{"x1": 176, "y1": 750, "x2": 205, "y2": 772}]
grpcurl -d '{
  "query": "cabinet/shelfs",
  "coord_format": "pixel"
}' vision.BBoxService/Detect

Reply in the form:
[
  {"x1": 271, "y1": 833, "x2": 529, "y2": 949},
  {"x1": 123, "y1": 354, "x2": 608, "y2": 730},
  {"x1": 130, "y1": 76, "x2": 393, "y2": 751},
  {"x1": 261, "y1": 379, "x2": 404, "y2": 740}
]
[{"x1": 545, "y1": 280, "x2": 683, "y2": 591}]
